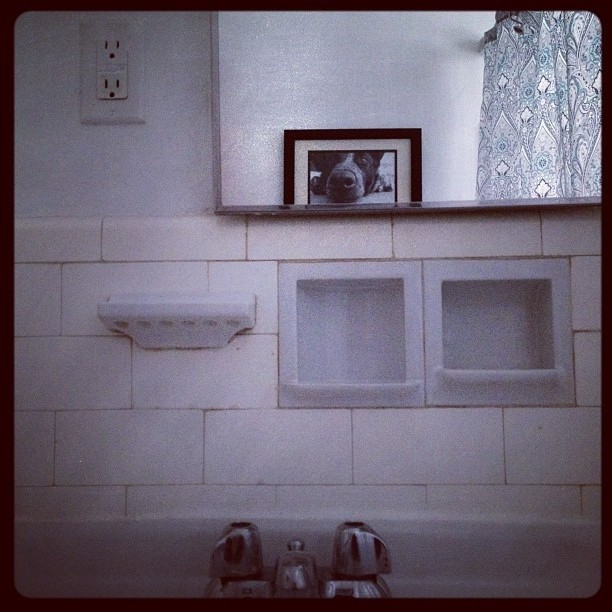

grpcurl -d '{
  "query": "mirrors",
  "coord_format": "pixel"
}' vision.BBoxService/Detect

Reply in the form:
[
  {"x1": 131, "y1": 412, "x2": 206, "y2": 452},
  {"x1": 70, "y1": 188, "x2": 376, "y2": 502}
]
[{"x1": 211, "y1": 9, "x2": 603, "y2": 215}]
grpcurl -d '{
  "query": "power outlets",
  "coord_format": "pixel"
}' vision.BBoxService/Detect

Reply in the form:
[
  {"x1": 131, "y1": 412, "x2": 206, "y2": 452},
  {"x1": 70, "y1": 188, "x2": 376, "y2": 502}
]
[{"x1": 78, "y1": 11, "x2": 148, "y2": 126}]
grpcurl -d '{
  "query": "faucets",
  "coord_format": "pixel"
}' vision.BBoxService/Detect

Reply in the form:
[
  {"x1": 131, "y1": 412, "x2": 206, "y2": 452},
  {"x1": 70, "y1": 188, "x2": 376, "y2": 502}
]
[{"x1": 271, "y1": 537, "x2": 320, "y2": 599}]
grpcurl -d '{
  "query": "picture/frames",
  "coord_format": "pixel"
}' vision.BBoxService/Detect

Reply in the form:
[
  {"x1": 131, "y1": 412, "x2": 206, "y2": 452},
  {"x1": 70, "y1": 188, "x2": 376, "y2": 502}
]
[{"x1": 283, "y1": 126, "x2": 423, "y2": 206}]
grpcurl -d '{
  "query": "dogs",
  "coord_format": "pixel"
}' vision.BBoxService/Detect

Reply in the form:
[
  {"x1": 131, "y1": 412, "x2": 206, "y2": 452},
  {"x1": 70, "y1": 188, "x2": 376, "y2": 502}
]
[{"x1": 310, "y1": 151, "x2": 392, "y2": 201}]
